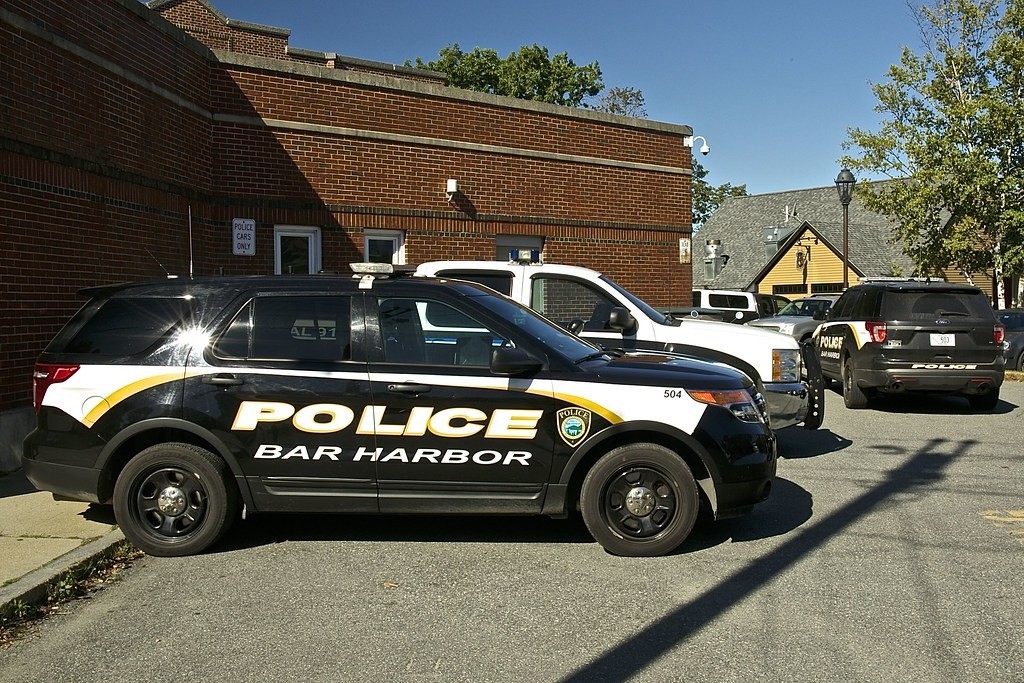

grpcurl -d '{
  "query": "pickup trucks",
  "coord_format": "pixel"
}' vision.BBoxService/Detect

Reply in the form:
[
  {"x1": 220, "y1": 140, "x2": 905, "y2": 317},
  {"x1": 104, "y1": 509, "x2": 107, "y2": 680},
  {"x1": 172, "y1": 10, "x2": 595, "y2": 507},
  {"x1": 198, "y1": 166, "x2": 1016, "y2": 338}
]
[{"x1": 411, "y1": 248, "x2": 811, "y2": 429}]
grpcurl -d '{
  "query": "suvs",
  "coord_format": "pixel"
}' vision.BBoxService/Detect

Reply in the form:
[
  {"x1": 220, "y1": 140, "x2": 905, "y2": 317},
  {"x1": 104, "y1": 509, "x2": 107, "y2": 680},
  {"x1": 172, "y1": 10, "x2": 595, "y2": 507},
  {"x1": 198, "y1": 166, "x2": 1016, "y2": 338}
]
[
  {"x1": 21, "y1": 261, "x2": 777, "y2": 561},
  {"x1": 813, "y1": 275, "x2": 1007, "y2": 411},
  {"x1": 692, "y1": 289, "x2": 844, "y2": 345}
]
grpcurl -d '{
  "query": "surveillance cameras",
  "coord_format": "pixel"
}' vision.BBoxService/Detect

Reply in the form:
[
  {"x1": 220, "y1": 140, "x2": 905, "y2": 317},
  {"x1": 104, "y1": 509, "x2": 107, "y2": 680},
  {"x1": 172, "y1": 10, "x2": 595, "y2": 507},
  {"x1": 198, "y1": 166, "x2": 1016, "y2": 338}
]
[{"x1": 700, "y1": 146, "x2": 710, "y2": 155}]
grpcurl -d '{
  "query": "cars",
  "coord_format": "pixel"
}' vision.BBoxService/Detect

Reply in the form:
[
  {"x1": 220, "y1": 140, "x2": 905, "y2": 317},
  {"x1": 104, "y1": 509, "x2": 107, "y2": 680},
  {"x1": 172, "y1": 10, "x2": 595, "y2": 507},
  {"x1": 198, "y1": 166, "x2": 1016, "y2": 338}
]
[{"x1": 994, "y1": 307, "x2": 1023, "y2": 372}]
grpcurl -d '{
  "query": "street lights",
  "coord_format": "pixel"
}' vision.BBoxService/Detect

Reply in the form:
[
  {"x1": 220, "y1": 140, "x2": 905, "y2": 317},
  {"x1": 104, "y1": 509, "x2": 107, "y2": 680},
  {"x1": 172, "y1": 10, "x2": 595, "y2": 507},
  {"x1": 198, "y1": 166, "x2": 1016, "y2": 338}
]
[{"x1": 833, "y1": 162, "x2": 858, "y2": 294}]
